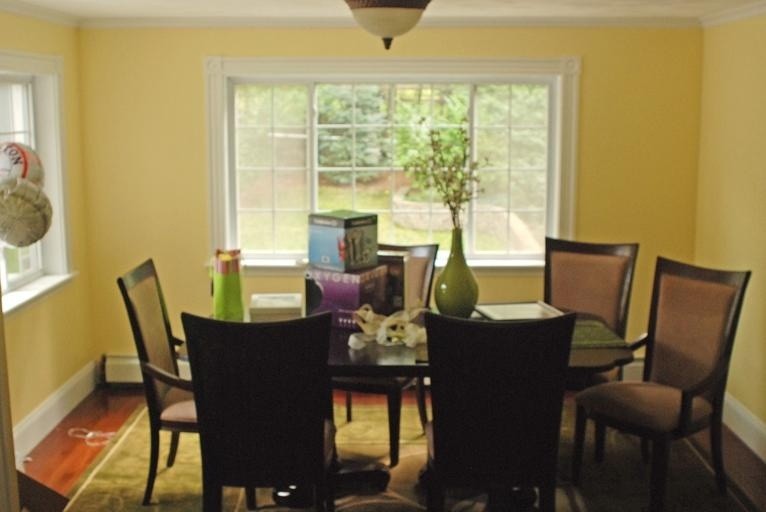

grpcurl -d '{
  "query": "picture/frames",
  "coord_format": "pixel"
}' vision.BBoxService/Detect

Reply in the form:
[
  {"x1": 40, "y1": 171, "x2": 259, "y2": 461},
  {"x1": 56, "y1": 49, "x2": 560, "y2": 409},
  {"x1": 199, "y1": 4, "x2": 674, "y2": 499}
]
[{"x1": 474, "y1": 299, "x2": 564, "y2": 321}]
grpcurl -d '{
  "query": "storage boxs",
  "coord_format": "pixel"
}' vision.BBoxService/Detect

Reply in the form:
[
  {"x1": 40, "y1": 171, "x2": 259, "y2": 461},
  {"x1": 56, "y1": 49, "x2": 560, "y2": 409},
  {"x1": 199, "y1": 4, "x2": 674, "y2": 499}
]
[
  {"x1": 309, "y1": 210, "x2": 378, "y2": 272},
  {"x1": 305, "y1": 266, "x2": 389, "y2": 327}
]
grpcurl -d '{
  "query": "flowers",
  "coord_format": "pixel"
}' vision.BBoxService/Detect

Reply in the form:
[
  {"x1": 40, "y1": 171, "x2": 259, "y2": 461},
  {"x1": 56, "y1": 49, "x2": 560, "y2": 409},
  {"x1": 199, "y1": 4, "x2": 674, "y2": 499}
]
[{"x1": 405, "y1": 112, "x2": 483, "y2": 228}]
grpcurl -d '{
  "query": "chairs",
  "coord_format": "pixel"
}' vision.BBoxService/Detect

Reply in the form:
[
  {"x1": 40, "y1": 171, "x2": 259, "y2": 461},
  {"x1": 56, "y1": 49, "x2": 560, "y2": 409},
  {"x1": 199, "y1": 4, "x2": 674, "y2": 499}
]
[
  {"x1": 567, "y1": 253, "x2": 752, "y2": 505},
  {"x1": 541, "y1": 237, "x2": 639, "y2": 461},
  {"x1": 181, "y1": 306, "x2": 389, "y2": 512},
  {"x1": 422, "y1": 308, "x2": 578, "y2": 511},
  {"x1": 333, "y1": 243, "x2": 443, "y2": 467},
  {"x1": 116, "y1": 257, "x2": 262, "y2": 509}
]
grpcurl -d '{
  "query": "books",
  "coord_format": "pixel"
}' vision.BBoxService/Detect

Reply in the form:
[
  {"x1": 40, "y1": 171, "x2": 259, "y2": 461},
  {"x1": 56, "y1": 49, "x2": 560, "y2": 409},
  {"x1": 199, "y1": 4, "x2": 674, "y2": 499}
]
[{"x1": 250, "y1": 293, "x2": 301, "y2": 321}]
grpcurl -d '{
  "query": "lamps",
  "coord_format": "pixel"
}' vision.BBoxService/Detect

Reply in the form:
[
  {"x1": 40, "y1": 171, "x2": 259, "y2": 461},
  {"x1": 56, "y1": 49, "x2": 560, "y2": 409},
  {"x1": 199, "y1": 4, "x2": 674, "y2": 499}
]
[{"x1": 335, "y1": 2, "x2": 437, "y2": 55}]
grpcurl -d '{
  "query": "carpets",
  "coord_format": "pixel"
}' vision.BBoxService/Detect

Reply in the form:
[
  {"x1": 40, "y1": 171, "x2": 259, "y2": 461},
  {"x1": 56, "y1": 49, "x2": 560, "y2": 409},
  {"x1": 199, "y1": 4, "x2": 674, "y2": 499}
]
[{"x1": 61, "y1": 401, "x2": 764, "y2": 512}]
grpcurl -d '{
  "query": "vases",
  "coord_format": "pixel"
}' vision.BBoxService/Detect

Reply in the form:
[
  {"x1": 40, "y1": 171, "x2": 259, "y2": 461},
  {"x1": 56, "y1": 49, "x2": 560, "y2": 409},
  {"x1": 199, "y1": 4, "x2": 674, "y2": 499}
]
[{"x1": 432, "y1": 225, "x2": 481, "y2": 321}]
